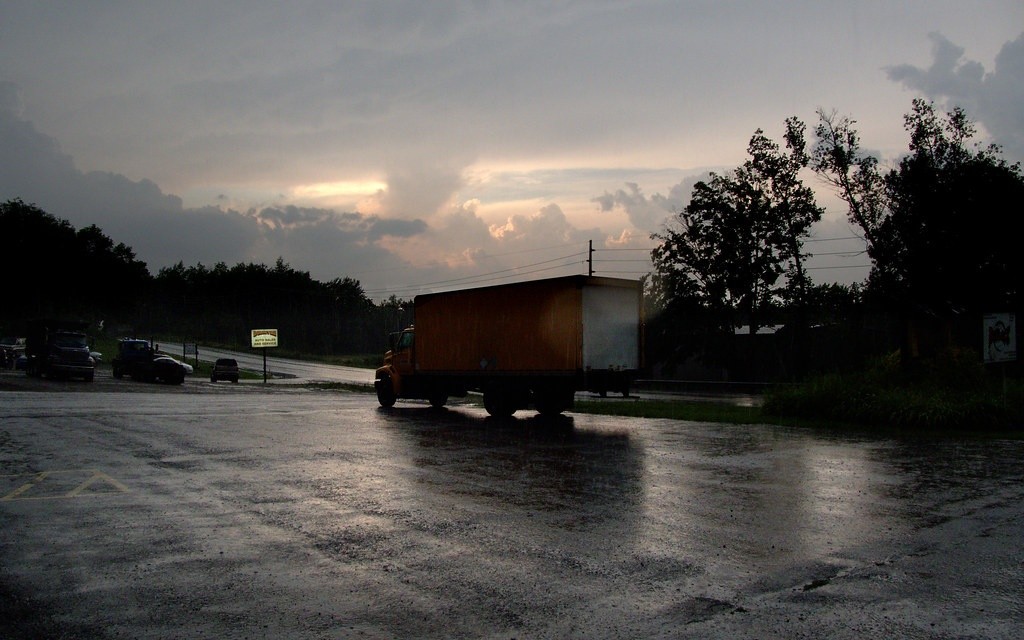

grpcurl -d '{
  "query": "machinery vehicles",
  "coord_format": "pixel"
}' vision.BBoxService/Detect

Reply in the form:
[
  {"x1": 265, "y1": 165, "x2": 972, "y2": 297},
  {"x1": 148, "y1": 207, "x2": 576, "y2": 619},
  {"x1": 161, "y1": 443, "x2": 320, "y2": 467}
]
[{"x1": 112, "y1": 336, "x2": 193, "y2": 384}]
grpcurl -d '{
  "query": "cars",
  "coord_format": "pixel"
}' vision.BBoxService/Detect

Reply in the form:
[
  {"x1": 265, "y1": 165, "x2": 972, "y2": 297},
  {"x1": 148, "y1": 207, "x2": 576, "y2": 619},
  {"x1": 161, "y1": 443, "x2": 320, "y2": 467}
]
[
  {"x1": 209, "y1": 358, "x2": 239, "y2": 383},
  {"x1": 0, "y1": 337, "x2": 27, "y2": 353},
  {"x1": 44, "y1": 331, "x2": 96, "y2": 382}
]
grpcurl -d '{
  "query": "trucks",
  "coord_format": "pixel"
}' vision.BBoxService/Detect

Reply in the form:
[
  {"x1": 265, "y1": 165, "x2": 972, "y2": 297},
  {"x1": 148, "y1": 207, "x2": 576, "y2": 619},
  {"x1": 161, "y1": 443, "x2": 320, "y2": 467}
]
[{"x1": 375, "y1": 276, "x2": 644, "y2": 416}]
